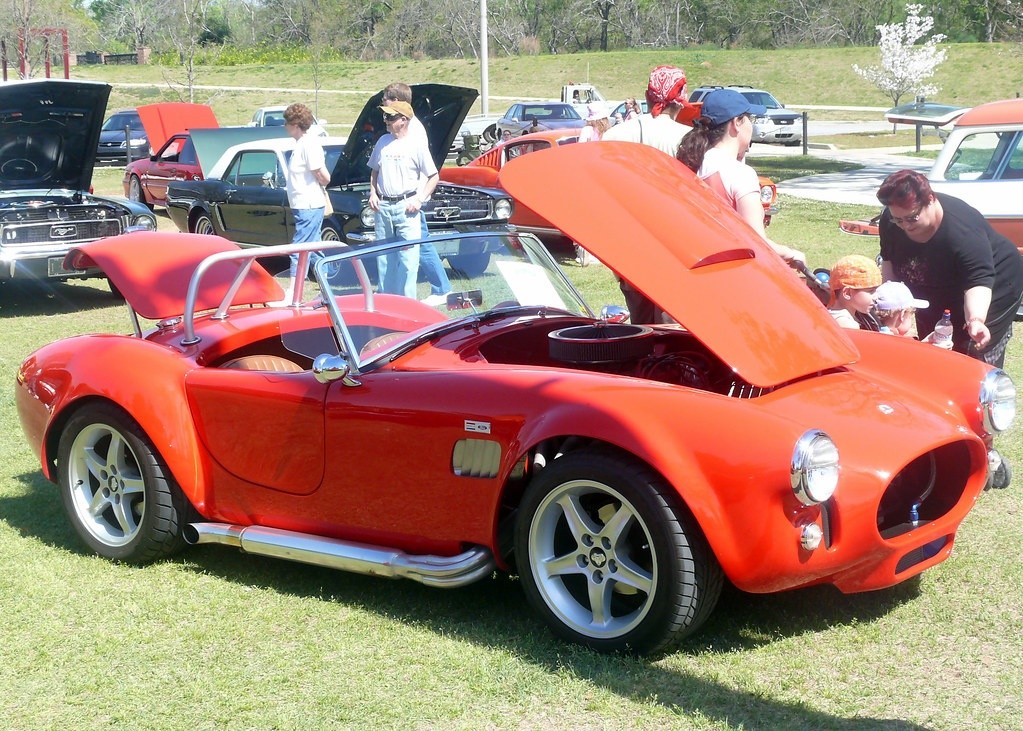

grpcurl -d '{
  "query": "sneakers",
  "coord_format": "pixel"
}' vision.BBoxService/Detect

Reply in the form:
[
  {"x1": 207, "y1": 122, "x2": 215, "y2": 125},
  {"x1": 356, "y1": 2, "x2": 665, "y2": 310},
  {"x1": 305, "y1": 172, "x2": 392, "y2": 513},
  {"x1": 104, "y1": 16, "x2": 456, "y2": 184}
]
[
  {"x1": 420, "y1": 291, "x2": 453, "y2": 306},
  {"x1": 577, "y1": 246, "x2": 590, "y2": 267}
]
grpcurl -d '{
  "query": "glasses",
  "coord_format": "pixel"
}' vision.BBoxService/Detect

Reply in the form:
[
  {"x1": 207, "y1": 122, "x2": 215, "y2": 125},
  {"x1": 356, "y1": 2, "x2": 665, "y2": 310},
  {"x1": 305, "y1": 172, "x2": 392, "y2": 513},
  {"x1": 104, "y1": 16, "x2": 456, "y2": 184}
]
[
  {"x1": 624, "y1": 102, "x2": 632, "y2": 104},
  {"x1": 284, "y1": 122, "x2": 296, "y2": 126},
  {"x1": 383, "y1": 112, "x2": 403, "y2": 121},
  {"x1": 889, "y1": 202, "x2": 923, "y2": 223}
]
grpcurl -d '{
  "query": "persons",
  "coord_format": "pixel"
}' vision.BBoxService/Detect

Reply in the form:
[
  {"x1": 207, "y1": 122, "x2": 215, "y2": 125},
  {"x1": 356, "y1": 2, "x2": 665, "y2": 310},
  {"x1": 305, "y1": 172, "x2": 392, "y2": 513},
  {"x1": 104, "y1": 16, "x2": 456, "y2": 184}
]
[
  {"x1": 874, "y1": 280, "x2": 929, "y2": 335},
  {"x1": 369, "y1": 102, "x2": 440, "y2": 300},
  {"x1": 830, "y1": 254, "x2": 882, "y2": 328},
  {"x1": 602, "y1": 66, "x2": 693, "y2": 324},
  {"x1": 612, "y1": 113, "x2": 623, "y2": 126},
  {"x1": 877, "y1": 170, "x2": 1023, "y2": 369},
  {"x1": 624, "y1": 97, "x2": 640, "y2": 121},
  {"x1": 678, "y1": 89, "x2": 807, "y2": 267},
  {"x1": 381, "y1": 83, "x2": 453, "y2": 307},
  {"x1": 498, "y1": 130, "x2": 529, "y2": 160},
  {"x1": 578, "y1": 101, "x2": 611, "y2": 142},
  {"x1": 267, "y1": 102, "x2": 331, "y2": 307}
]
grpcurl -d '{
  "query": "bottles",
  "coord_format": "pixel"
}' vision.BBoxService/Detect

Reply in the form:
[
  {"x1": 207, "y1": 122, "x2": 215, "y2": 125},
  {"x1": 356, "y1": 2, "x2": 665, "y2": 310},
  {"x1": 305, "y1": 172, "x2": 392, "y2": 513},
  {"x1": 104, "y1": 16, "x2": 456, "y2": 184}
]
[{"x1": 933, "y1": 309, "x2": 953, "y2": 350}]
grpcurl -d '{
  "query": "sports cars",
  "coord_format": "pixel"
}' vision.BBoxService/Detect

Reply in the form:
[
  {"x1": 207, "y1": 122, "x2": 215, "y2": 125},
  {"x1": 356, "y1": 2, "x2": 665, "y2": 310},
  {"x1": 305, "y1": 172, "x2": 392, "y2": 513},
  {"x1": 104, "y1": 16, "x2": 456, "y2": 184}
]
[{"x1": 8, "y1": 138, "x2": 1021, "y2": 664}]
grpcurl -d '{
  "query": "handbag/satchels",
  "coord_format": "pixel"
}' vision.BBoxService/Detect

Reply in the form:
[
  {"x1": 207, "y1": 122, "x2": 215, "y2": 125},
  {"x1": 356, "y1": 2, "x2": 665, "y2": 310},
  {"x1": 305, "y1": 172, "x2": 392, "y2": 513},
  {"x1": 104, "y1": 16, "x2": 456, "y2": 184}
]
[{"x1": 323, "y1": 187, "x2": 334, "y2": 219}]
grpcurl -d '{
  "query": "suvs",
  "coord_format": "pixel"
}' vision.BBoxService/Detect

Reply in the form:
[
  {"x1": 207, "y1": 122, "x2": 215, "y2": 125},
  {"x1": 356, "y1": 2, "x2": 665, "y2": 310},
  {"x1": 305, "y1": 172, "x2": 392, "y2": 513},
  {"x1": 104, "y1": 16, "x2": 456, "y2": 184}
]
[{"x1": 688, "y1": 83, "x2": 804, "y2": 148}]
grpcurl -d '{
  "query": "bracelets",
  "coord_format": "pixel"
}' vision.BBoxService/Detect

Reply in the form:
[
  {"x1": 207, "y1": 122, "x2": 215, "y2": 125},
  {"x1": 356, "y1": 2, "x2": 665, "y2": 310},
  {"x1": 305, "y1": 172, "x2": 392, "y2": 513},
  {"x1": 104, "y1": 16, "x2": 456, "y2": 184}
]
[{"x1": 963, "y1": 318, "x2": 984, "y2": 331}]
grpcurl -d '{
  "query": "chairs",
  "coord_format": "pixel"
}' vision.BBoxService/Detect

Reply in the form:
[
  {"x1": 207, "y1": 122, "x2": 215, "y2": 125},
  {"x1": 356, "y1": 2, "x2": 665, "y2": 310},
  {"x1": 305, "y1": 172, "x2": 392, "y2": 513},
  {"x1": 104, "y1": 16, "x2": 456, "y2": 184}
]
[{"x1": 216, "y1": 355, "x2": 304, "y2": 372}]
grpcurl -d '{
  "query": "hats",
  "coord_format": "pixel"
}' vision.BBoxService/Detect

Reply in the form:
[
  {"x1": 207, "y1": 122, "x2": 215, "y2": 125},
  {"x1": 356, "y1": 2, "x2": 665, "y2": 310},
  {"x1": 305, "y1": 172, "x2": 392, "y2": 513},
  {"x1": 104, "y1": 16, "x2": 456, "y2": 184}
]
[
  {"x1": 376, "y1": 102, "x2": 414, "y2": 119},
  {"x1": 872, "y1": 280, "x2": 929, "y2": 310},
  {"x1": 585, "y1": 101, "x2": 612, "y2": 120},
  {"x1": 701, "y1": 89, "x2": 767, "y2": 124}
]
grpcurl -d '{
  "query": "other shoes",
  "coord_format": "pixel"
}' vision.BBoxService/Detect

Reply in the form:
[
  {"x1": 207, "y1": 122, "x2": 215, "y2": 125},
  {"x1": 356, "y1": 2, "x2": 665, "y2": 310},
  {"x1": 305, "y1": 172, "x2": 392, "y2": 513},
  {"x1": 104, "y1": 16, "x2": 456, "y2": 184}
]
[
  {"x1": 265, "y1": 292, "x2": 295, "y2": 308},
  {"x1": 313, "y1": 293, "x2": 328, "y2": 303}
]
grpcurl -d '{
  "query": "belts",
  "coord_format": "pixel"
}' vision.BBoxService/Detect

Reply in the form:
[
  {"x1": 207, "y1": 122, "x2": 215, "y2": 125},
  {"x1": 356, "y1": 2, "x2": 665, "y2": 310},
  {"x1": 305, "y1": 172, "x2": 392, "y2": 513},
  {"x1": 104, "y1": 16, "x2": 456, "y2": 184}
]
[{"x1": 377, "y1": 191, "x2": 416, "y2": 201}]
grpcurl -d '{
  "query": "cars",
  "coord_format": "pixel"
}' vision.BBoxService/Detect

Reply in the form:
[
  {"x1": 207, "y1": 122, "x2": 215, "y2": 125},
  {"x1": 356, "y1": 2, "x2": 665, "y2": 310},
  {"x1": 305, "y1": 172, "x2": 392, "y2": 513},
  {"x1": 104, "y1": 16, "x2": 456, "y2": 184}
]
[
  {"x1": 838, "y1": 96, "x2": 1022, "y2": 320},
  {"x1": 120, "y1": 82, "x2": 703, "y2": 276},
  {"x1": 0, "y1": 77, "x2": 159, "y2": 292},
  {"x1": 93, "y1": 110, "x2": 153, "y2": 165}
]
